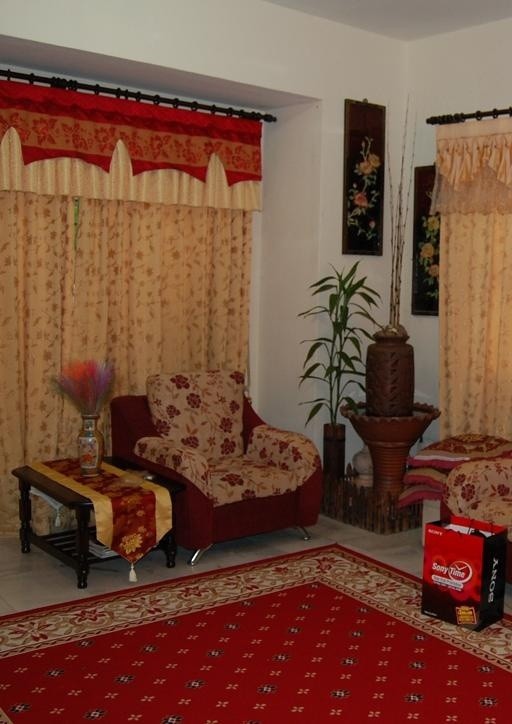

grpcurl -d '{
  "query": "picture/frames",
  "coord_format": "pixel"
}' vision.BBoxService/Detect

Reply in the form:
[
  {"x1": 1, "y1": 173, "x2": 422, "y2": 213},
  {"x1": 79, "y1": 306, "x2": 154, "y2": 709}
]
[
  {"x1": 342, "y1": 97, "x2": 389, "y2": 254},
  {"x1": 411, "y1": 164, "x2": 440, "y2": 317}
]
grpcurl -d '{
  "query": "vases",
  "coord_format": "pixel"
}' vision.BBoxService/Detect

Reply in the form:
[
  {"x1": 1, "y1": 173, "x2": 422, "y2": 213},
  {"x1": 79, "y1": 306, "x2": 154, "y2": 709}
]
[
  {"x1": 337, "y1": 402, "x2": 443, "y2": 495},
  {"x1": 76, "y1": 413, "x2": 105, "y2": 476},
  {"x1": 364, "y1": 332, "x2": 416, "y2": 417}
]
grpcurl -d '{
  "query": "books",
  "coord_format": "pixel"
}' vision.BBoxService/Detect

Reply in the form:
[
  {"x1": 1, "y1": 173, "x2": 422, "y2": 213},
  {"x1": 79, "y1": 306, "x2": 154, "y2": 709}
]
[{"x1": 89, "y1": 538, "x2": 122, "y2": 559}]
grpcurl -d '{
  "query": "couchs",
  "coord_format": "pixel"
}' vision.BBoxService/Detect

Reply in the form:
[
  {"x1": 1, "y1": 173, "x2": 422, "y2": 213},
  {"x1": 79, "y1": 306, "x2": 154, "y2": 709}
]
[
  {"x1": 110, "y1": 370, "x2": 323, "y2": 568},
  {"x1": 443, "y1": 457, "x2": 512, "y2": 589}
]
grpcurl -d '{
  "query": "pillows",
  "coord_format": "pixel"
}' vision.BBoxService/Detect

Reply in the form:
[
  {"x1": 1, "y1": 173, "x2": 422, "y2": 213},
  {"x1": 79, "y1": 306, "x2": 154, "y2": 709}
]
[{"x1": 398, "y1": 434, "x2": 512, "y2": 509}]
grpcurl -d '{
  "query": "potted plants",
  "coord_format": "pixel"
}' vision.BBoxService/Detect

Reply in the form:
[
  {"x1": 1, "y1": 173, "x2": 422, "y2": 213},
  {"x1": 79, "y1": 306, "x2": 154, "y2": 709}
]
[{"x1": 298, "y1": 263, "x2": 385, "y2": 481}]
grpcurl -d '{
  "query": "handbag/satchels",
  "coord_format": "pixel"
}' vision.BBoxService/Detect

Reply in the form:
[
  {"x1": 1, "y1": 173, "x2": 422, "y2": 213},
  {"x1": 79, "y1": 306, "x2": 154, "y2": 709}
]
[{"x1": 421, "y1": 515, "x2": 508, "y2": 633}]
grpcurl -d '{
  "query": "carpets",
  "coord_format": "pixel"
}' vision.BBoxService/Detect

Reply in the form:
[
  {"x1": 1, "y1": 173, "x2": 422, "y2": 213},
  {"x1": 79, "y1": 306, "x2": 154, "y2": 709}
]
[{"x1": 0, "y1": 542, "x2": 511, "y2": 723}]
